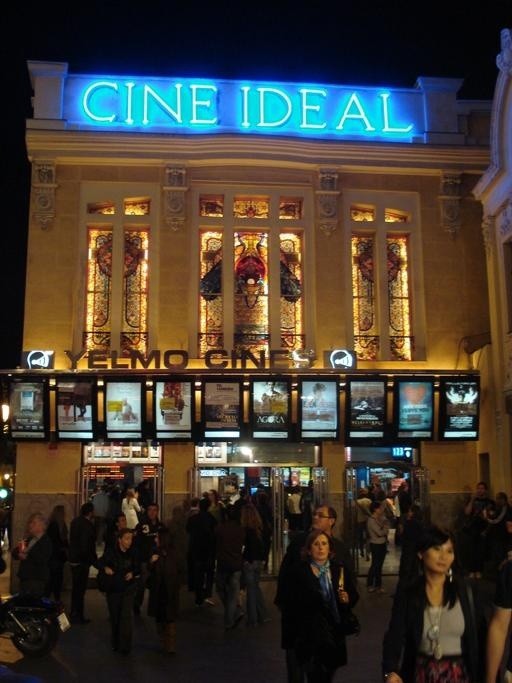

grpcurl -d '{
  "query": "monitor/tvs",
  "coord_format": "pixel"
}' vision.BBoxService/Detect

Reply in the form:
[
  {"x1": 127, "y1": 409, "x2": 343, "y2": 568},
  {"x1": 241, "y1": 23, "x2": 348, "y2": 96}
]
[
  {"x1": 250, "y1": 487, "x2": 258, "y2": 496},
  {"x1": 200, "y1": 468, "x2": 229, "y2": 477}
]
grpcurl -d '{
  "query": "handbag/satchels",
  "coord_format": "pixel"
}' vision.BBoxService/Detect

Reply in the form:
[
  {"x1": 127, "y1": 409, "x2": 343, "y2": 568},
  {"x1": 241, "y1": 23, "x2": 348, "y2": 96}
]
[{"x1": 339, "y1": 589, "x2": 362, "y2": 636}]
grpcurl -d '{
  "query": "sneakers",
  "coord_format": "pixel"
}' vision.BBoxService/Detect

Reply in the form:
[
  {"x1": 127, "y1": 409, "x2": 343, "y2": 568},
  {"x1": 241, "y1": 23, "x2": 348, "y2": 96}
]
[
  {"x1": 70, "y1": 618, "x2": 94, "y2": 625},
  {"x1": 110, "y1": 645, "x2": 133, "y2": 659},
  {"x1": 367, "y1": 587, "x2": 388, "y2": 595},
  {"x1": 222, "y1": 612, "x2": 272, "y2": 633},
  {"x1": 203, "y1": 596, "x2": 218, "y2": 608}
]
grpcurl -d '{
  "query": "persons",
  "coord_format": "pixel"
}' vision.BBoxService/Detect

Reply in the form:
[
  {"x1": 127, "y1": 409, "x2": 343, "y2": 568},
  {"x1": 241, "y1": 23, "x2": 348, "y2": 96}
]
[
  {"x1": 1, "y1": 475, "x2": 512, "y2": 683},
  {"x1": 175, "y1": 395, "x2": 185, "y2": 419},
  {"x1": 114, "y1": 396, "x2": 132, "y2": 423},
  {"x1": 303, "y1": 383, "x2": 331, "y2": 421},
  {"x1": 457, "y1": 390, "x2": 466, "y2": 403},
  {"x1": 260, "y1": 381, "x2": 283, "y2": 416}
]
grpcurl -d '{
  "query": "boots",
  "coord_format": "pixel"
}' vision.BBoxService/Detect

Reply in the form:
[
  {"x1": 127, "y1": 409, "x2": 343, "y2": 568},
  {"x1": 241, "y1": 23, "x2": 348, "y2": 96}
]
[{"x1": 157, "y1": 622, "x2": 177, "y2": 654}]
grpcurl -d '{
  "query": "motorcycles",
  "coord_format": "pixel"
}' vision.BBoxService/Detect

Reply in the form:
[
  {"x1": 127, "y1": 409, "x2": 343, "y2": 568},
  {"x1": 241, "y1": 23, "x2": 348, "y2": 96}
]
[{"x1": 0, "y1": 593, "x2": 71, "y2": 659}]
